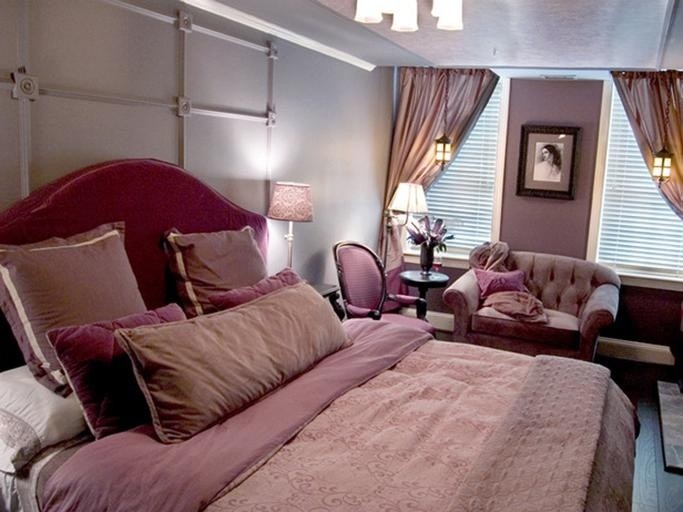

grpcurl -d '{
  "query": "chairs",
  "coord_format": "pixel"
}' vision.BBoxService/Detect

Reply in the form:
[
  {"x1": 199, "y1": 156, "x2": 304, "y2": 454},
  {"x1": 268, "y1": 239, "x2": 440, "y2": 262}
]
[{"x1": 332, "y1": 237, "x2": 429, "y2": 325}]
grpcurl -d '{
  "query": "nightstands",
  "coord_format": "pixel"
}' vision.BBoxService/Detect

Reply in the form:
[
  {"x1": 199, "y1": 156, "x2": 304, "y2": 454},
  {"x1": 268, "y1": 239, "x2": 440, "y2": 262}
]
[{"x1": 304, "y1": 277, "x2": 346, "y2": 320}]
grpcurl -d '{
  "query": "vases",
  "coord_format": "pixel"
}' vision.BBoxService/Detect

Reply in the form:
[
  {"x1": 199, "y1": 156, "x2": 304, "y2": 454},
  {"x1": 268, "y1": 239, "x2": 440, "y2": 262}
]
[{"x1": 418, "y1": 244, "x2": 435, "y2": 276}]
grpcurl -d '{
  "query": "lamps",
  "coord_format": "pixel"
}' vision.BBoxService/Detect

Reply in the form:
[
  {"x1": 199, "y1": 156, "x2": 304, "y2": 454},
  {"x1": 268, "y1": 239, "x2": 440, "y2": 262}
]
[
  {"x1": 387, "y1": 181, "x2": 426, "y2": 227},
  {"x1": 650, "y1": 73, "x2": 674, "y2": 188},
  {"x1": 352, "y1": 0, "x2": 463, "y2": 34},
  {"x1": 265, "y1": 180, "x2": 314, "y2": 271},
  {"x1": 432, "y1": 71, "x2": 452, "y2": 172}
]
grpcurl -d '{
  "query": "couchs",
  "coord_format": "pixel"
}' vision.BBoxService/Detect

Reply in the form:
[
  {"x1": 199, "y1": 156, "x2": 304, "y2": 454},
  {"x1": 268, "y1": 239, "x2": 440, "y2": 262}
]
[{"x1": 441, "y1": 250, "x2": 621, "y2": 361}]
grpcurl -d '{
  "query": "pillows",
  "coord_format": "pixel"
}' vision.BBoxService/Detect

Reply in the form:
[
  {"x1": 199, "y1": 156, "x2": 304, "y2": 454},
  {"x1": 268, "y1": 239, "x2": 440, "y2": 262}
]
[
  {"x1": 475, "y1": 269, "x2": 526, "y2": 295},
  {"x1": 45, "y1": 302, "x2": 188, "y2": 440},
  {"x1": 0, "y1": 220, "x2": 147, "y2": 397},
  {"x1": 161, "y1": 223, "x2": 268, "y2": 318},
  {"x1": 112, "y1": 280, "x2": 352, "y2": 445},
  {"x1": 1, "y1": 365, "x2": 85, "y2": 475},
  {"x1": 205, "y1": 267, "x2": 304, "y2": 310}
]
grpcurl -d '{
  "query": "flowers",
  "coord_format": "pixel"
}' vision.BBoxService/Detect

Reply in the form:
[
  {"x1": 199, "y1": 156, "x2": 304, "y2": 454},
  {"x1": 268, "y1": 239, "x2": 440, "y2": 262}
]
[{"x1": 407, "y1": 215, "x2": 454, "y2": 252}]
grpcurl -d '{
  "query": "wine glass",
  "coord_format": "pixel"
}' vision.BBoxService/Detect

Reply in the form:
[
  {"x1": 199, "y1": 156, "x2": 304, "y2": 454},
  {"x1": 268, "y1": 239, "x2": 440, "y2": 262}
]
[{"x1": 432, "y1": 260, "x2": 442, "y2": 278}]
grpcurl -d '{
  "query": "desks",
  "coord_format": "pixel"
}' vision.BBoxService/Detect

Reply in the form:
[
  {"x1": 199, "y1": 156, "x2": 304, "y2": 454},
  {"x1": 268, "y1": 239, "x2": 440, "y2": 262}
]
[{"x1": 399, "y1": 270, "x2": 449, "y2": 322}]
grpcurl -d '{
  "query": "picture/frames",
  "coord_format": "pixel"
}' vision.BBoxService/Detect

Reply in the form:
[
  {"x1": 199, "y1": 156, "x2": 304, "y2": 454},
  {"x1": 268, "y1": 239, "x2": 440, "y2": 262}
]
[{"x1": 513, "y1": 124, "x2": 582, "y2": 201}]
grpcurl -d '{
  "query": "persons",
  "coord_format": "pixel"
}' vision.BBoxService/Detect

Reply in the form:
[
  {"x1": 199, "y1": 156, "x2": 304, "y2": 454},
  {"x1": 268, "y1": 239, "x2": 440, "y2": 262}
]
[{"x1": 532, "y1": 143, "x2": 562, "y2": 182}]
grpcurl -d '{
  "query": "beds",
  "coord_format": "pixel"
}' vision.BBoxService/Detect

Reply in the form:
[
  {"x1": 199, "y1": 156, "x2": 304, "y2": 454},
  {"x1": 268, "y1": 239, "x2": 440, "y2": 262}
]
[{"x1": 1, "y1": 157, "x2": 640, "y2": 512}]
